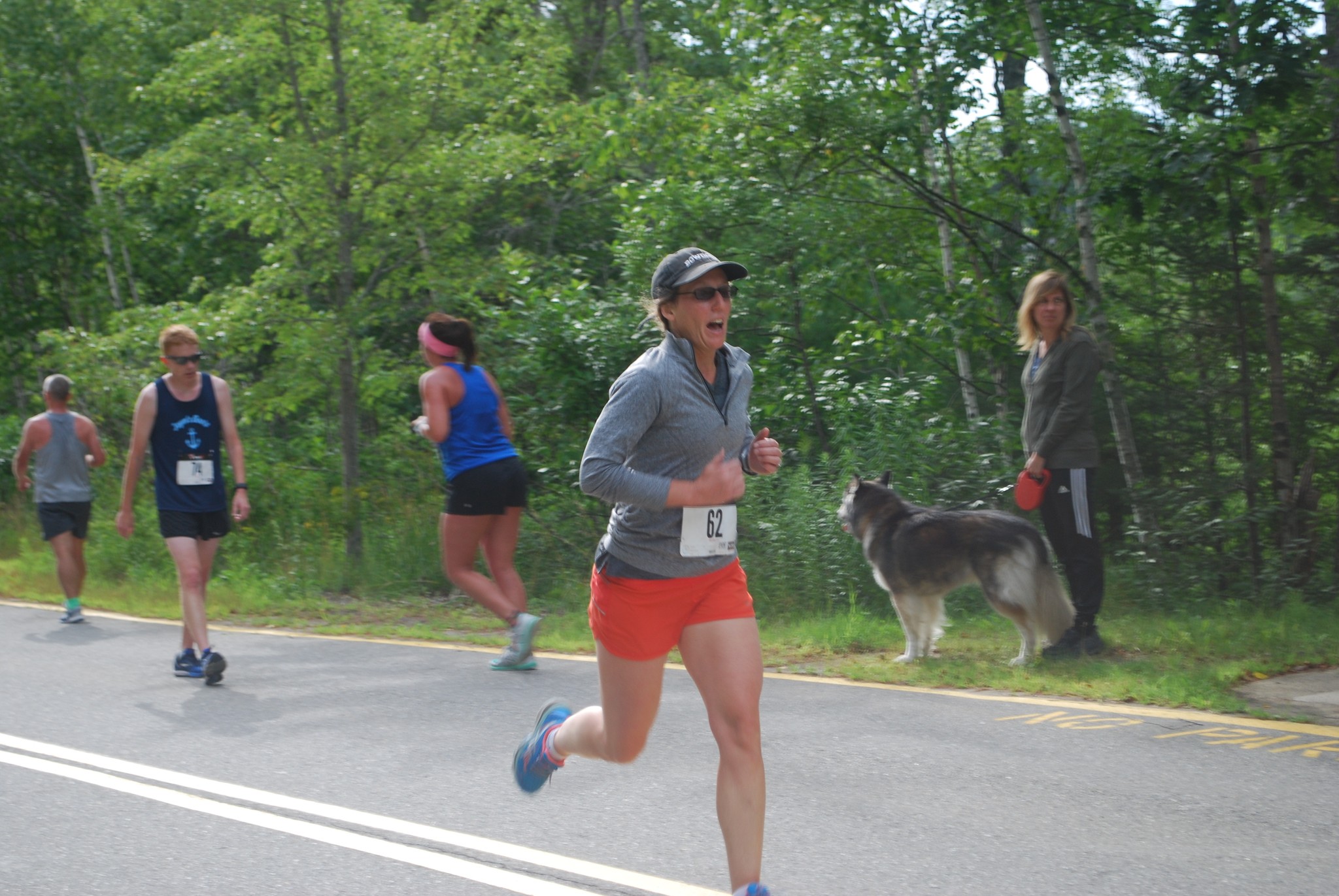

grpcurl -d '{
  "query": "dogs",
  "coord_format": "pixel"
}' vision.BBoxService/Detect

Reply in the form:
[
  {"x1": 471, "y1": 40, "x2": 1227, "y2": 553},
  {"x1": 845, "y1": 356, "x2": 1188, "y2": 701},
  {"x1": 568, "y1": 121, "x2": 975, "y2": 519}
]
[{"x1": 837, "y1": 468, "x2": 1081, "y2": 668}]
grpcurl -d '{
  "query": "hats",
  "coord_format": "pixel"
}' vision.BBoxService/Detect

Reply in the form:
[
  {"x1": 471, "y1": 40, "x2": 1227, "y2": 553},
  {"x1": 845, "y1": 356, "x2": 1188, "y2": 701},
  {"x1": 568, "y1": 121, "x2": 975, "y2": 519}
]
[{"x1": 651, "y1": 246, "x2": 750, "y2": 299}]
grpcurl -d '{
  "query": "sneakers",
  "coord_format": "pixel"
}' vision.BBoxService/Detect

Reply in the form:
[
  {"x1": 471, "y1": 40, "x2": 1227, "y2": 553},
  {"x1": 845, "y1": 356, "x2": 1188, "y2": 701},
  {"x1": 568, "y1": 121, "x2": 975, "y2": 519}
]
[
  {"x1": 200, "y1": 646, "x2": 226, "y2": 686},
  {"x1": 511, "y1": 697, "x2": 575, "y2": 793},
  {"x1": 508, "y1": 612, "x2": 544, "y2": 662},
  {"x1": 174, "y1": 648, "x2": 205, "y2": 678},
  {"x1": 61, "y1": 606, "x2": 85, "y2": 624},
  {"x1": 729, "y1": 880, "x2": 770, "y2": 896},
  {"x1": 487, "y1": 645, "x2": 539, "y2": 671},
  {"x1": 1042, "y1": 624, "x2": 1107, "y2": 661}
]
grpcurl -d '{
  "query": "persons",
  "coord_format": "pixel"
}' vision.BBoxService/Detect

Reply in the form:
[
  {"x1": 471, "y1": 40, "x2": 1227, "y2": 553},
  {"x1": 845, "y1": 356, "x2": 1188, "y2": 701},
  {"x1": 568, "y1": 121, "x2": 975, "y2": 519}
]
[
  {"x1": 13, "y1": 374, "x2": 107, "y2": 622},
  {"x1": 1012, "y1": 269, "x2": 1126, "y2": 661},
  {"x1": 115, "y1": 323, "x2": 252, "y2": 685},
  {"x1": 513, "y1": 248, "x2": 782, "y2": 896},
  {"x1": 408, "y1": 312, "x2": 543, "y2": 669}
]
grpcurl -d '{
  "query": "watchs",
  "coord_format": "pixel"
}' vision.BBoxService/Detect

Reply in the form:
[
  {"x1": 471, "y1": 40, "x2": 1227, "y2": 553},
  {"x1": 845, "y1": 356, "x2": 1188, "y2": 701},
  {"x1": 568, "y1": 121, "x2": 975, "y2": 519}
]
[{"x1": 233, "y1": 483, "x2": 248, "y2": 490}]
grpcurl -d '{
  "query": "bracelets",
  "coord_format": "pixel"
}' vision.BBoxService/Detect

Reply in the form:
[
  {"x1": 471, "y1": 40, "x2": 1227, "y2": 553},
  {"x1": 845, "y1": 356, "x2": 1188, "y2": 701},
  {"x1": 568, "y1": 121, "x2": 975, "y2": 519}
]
[{"x1": 412, "y1": 422, "x2": 427, "y2": 433}]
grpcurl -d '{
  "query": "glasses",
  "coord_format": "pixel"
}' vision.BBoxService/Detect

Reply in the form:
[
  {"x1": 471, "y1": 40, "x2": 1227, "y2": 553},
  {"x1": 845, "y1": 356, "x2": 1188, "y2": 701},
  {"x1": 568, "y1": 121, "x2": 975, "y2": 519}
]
[
  {"x1": 164, "y1": 352, "x2": 204, "y2": 365},
  {"x1": 674, "y1": 285, "x2": 740, "y2": 301}
]
